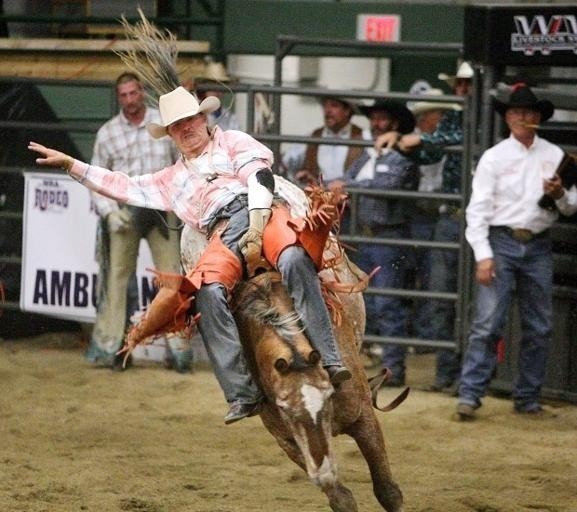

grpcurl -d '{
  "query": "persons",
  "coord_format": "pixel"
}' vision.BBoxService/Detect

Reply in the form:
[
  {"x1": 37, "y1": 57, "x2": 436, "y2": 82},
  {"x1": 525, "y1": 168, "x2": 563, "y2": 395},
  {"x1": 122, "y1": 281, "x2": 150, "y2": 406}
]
[
  {"x1": 90, "y1": 73, "x2": 192, "y2": 372},
  {"x1": 330, "y1": 62, "x2": 505, "y2": 394},
  {"x1": 26, "y1": 86, "x2": 352, "y2": 424},
  {"x1": 278, "y1": 94, "x2": 372, "y2": 234},
  {"x1": 457, "y1": 88, "x2": 577, "y2": 420}
]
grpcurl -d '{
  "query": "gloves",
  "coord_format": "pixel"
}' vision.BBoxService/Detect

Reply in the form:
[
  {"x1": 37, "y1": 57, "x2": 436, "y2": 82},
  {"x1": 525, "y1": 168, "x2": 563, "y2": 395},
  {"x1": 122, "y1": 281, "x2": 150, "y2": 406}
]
[
  {"x1": 236, "y1": 209, "x2": 271, "y2": 258},
  {"x1": 106, "y1": 210, "x2": 130, "y2": 234}
]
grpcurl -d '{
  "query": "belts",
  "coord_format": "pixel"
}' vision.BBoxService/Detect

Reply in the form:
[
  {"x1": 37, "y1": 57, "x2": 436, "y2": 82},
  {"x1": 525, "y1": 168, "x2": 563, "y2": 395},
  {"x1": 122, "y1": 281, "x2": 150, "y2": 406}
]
[{"x1": 503, "y1": 227, "x2": 545, "y2": 243}]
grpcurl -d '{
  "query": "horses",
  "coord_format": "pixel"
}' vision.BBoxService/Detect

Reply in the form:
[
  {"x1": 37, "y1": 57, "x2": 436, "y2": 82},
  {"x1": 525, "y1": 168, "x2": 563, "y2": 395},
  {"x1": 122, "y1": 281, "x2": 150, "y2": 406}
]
[{"x1": 105, "y1": 5, "x2": 405, "y2": 512}]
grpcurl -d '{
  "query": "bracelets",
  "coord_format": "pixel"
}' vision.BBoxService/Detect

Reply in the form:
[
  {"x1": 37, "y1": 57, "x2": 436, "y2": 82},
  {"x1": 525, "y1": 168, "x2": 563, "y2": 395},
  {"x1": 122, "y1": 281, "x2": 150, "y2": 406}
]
[{"x1": 61, "y1": 156, "x2": 70, "y2": 170}]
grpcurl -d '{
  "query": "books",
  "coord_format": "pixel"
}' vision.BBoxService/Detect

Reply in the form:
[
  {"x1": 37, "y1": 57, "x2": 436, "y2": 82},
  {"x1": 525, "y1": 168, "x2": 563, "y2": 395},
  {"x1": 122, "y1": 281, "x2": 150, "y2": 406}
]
[{"x1": 537, "y1": 152, "x2": 577, "y2": 212}]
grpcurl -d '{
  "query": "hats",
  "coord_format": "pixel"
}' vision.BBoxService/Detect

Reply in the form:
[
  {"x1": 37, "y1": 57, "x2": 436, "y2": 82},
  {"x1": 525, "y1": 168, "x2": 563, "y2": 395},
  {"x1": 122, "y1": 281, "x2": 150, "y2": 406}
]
[
  {"x1": 489, "y1": 86, "x2": 553, "y2": 123},
  {"x1": 316, "y1": 61, "x2": 483, "y2": 135},
  {"x1": 146, "y1": 86, "x2": 220, "y2": 139}
]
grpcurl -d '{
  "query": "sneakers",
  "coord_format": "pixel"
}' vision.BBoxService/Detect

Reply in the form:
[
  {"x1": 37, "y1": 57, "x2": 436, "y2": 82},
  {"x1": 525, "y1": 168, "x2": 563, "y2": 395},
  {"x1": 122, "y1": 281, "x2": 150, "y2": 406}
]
[
  {"x1": 329, "y1": 365, "x2": 351, "y2": 389},
  {"x1": 520, "y1": 403, "x2": 557, "y2": 421},
  {"x1": 223, "y1": 397, "x2": 263, "y2": 425},
  {"x1": 457, "y1": 402, "x2": 474, "y2": 416}
]
